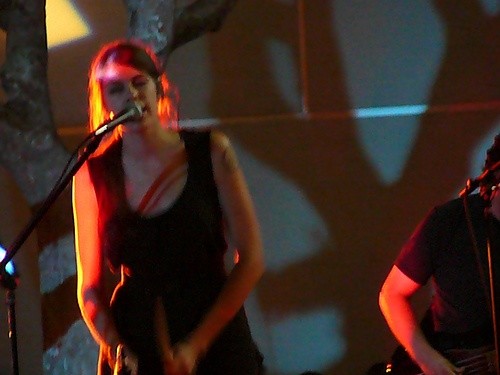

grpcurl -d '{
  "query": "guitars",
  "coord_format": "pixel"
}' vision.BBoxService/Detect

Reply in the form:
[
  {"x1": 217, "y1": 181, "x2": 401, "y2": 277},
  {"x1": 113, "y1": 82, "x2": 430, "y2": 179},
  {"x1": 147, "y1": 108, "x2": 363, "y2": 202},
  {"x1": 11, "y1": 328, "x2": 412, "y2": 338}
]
[{"x1": 391, "y1": 331, "x2": 500, "y2": 375}]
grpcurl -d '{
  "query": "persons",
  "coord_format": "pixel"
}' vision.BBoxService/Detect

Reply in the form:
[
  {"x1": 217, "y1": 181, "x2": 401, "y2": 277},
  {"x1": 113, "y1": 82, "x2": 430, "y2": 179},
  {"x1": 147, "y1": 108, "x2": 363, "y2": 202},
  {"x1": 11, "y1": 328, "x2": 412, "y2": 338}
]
[
  {"x1": 72, "y1": 37, "x2": 266, "y2": 375},
  {"x1": 378, "y1": 132, "x2": 500, "y2": 375}
]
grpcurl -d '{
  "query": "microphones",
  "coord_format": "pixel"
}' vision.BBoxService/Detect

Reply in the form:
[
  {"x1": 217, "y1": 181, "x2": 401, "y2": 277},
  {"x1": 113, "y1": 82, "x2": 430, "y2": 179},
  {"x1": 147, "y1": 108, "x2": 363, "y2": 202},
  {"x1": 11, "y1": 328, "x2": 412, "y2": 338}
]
[
  {"x1": 460, "y1": 160, "x2": 500, "y2": 196},
  {"x1": 90, "y1": 103, "x2": 143, "y2": 137}
]
